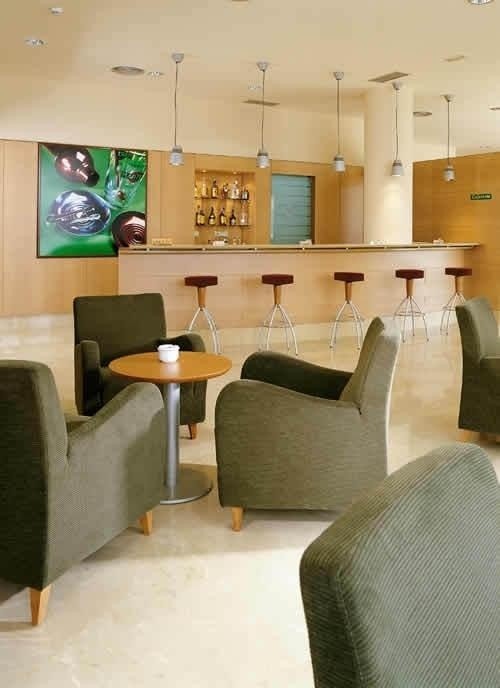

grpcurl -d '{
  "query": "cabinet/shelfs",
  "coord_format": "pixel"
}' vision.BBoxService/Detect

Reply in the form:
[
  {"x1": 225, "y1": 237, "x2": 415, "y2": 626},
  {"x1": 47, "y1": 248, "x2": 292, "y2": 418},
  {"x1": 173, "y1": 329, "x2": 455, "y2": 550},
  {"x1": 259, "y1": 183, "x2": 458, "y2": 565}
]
[{"x1": 194, "y1": 197, "x2": 251, "y2": 227}]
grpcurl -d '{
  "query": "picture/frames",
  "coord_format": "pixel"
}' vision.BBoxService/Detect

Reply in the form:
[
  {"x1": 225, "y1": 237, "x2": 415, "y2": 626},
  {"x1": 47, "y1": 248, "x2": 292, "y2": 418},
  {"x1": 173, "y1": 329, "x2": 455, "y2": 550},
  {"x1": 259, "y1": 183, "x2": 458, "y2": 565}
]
[{"x1": 36, "y1": 142, "x2": 148, "y2": 259}]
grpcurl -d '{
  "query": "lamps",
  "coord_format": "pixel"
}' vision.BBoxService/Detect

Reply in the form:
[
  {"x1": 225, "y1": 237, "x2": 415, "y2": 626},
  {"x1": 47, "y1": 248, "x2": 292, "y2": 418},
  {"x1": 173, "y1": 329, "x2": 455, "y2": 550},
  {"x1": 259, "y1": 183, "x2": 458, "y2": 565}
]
[{"x1": 169, "y1": 54, "x2": 456, "y2": 183}]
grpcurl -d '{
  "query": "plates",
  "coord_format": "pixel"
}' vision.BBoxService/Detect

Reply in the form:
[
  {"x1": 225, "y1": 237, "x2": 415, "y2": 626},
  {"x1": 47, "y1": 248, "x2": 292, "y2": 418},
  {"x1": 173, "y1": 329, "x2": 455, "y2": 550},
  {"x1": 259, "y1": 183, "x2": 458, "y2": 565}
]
[{"x1": 49, "y1": 190, "x2": 111, "y2": 236}]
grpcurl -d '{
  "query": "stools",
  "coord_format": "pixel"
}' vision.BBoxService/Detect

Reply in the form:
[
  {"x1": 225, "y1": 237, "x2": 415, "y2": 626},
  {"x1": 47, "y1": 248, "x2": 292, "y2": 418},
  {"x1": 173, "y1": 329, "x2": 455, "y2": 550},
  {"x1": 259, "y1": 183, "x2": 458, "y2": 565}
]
[{"x1": 184, "y1": 267, "x2": 473, "y2": 354}]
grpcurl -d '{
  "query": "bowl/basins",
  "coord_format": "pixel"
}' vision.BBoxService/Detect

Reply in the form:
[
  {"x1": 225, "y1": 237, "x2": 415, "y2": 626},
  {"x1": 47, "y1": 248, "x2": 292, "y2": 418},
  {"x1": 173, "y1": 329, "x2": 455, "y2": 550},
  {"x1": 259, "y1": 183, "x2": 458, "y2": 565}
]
[
  {"x1": 110, "y1": 210, "x2": 146, "y2": 251},
  {"x1": 157, "y1": 344, "x2": 180, "y2": 363}
]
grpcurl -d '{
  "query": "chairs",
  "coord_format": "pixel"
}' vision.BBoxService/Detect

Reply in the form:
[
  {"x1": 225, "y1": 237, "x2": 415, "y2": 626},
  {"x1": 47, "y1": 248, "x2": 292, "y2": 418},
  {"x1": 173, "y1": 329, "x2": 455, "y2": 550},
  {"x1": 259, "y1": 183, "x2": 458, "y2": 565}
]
[
  {"x1": 455, "y1": 294, "x2": 499, "y2": 440},
  {"x1": 299, "y1": 440, "x2": 500, "y2": 688},
  {"x1": 214, "y1": 315, "x2": 402, "y2": 531},
  {"x1": 0, "y1": 359, "x2": 169, "y2": 626},
  {"x1": 72, "y1": 292, "x2": 207, "y2": 440}
]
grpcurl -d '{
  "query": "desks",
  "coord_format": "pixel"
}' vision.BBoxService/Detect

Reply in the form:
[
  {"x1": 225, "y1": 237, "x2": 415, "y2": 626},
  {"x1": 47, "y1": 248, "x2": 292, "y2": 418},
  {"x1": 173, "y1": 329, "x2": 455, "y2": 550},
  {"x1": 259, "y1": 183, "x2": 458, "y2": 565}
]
[{"x1": 107, "y1": 351, "x2": 232, "y2": 505}]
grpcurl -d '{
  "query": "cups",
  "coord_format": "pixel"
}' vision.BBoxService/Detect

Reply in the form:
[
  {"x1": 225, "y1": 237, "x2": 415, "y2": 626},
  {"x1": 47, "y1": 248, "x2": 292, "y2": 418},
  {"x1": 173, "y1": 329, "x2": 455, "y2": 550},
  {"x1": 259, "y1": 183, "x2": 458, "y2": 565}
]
[{"x1": 98, "y1": 150, "x2": 147, "y2": 210}]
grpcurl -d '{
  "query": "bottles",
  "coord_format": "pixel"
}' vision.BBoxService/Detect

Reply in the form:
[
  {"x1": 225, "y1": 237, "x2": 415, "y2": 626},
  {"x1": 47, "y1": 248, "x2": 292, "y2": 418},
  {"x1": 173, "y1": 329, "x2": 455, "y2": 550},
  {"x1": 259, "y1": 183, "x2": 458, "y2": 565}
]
[
  {"x1": 192, "y1": 171, "x2": 250, "y2": 227},
  {"x1": 231, "y1": 235, "x2": 242, "y2": 245},
  {"x1": 54, "y1": 147, "x2": 100, "y2": 187}
]
